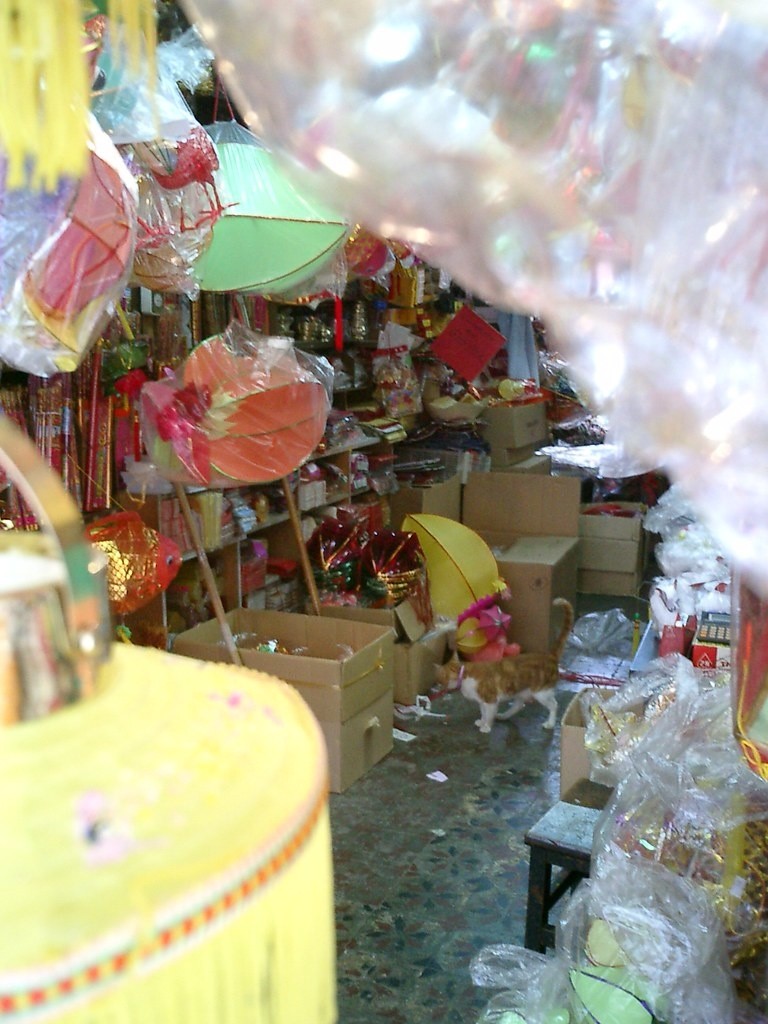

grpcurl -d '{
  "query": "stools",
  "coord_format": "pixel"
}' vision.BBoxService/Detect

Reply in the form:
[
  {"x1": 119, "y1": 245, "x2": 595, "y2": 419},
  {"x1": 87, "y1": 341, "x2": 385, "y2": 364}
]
[{"x1": 522, "y1": 779, "x2": 614, "y2": 956}]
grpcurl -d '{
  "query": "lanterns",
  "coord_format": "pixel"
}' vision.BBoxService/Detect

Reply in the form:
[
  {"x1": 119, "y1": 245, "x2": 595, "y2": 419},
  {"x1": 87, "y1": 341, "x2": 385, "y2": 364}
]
[
  {"x1": 193, "y1": 143, "x2": 351, "y2": 299},
  {"x1": 400, "y1": 511, "x2": 523, "y2": 663},
  {"x1": 0, "y1": 54, "x2": 218, "y2": 379},
  {"x1": 138, "y1": 329, "x2": 332, "y2": 491}
]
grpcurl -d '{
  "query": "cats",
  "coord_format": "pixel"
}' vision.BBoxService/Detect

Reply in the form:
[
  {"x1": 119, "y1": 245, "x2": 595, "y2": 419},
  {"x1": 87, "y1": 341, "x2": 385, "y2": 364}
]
[{"x1": 432, "y1": 597, "x2": 575, "y2": 733}]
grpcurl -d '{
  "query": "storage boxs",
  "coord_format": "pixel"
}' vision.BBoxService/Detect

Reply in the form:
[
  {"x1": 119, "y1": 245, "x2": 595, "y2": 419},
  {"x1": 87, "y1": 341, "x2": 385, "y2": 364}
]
[
  {"x1": 493, "y1": 455, "x2": 551, "y2": 474},
  {"x1": 579, "y1": 503, "x2": 651, "y2": 596},
  {"x1": 479, "y1": 401, "x2": 547, "y2": 466},
  {"x1": 559, "y1": 687, "x2": 617, "y2": 810},
  {"x1": 421, "y1": 471, "x2": 581, "y2": 655},
  {"x1": 171, "y1": 607, "x2": 393, "y2": 794},
  {"x1": 305, "y1": 596, "x2": 427, "y2": 642},
  {"x1": 394, "y1": 630, "x2": 448, "y2": 706}
]
